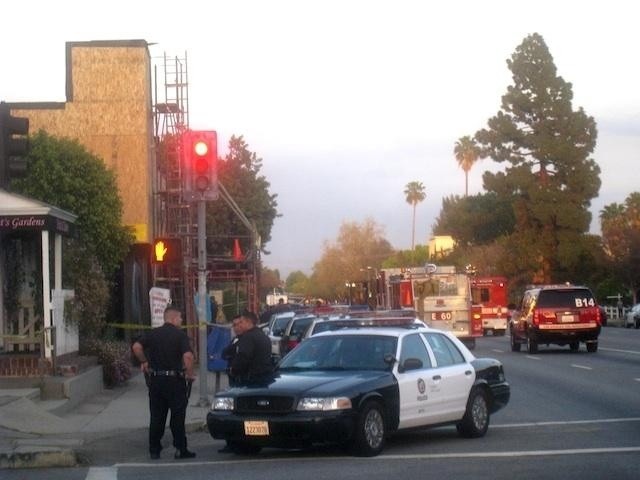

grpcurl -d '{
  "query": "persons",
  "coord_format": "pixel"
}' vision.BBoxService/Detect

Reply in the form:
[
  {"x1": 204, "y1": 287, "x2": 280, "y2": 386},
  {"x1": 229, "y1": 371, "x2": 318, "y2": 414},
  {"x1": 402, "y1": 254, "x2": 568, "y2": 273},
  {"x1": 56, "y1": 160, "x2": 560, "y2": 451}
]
[
  {"x1": 217, "y1": 315, "x2": 242, "y2": 453},
  {"x1": 229, "y1": 311, "x2": 273, "y2": 385},
  {"x1": 617, "y1": 298, "x2": 623, "y2": 318},
  {"x1": 132, "y1": 305, "x2": 196, "y2": 461}
]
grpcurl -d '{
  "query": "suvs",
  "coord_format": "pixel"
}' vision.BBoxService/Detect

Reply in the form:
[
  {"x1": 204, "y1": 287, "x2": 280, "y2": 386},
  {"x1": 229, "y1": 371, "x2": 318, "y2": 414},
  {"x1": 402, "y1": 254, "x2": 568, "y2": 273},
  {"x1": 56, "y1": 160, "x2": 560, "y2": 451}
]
[{"x1": 508, "y1": 281, "x2": 601, "y2": 351}]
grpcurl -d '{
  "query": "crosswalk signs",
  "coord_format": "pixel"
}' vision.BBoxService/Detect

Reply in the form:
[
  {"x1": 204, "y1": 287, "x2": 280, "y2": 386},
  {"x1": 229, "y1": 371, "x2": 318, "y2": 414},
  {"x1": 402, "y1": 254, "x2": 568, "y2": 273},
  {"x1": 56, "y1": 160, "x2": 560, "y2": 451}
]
[{"x1": 152, "y1": 236, "x2": 183, "y2": 263}]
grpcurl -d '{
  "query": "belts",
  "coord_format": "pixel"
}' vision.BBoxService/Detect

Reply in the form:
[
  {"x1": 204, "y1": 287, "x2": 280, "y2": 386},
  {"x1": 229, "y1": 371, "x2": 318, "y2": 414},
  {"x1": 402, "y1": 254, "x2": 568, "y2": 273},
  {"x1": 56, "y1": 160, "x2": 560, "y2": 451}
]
[{"x1": 150, "y1": 370, "x2": 185, "y2": 377}]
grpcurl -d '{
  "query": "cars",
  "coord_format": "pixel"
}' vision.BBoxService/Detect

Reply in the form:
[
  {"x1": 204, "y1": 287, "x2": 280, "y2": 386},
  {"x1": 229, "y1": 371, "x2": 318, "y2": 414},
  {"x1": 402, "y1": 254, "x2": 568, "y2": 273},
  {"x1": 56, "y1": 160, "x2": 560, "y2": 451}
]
[
  {"x1": 625, "y1": 304, "x2": 639, "y2": 327},
  {"x1": 207, "y1": 326, "x2": 509, "y2": 457},
  {"x1": 264, "y1": 304, "x2": 428, "y2": 357}
]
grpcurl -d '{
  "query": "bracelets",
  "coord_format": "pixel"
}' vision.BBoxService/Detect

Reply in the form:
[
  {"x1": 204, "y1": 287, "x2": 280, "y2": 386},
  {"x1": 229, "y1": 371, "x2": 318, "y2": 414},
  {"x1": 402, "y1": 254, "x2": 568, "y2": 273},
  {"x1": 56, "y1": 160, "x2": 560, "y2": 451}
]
[{"x1": 140, "y1": 360, "x2": 148, "y2": 364}]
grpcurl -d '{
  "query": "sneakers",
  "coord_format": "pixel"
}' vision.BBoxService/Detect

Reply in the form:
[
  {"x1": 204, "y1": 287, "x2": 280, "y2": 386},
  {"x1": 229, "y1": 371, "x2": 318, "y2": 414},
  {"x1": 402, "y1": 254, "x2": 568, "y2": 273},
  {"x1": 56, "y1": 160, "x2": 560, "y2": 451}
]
[
  {"x1": 174, "y1": 449, "x2": 196, "y2": 459},
  {"x1": 217, "y1": 445, "x2": 233, "y2": 454},
  {"x1": 150, "y1": 452, "x2": 160, "y2": 460}
]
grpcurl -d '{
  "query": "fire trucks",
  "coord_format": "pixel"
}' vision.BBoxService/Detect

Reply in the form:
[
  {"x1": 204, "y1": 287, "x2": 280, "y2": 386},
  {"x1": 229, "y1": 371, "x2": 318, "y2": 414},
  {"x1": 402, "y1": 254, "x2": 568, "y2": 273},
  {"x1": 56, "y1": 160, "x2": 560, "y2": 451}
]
[{"x1": 372, "y1": 264, "x2": 509, "y2": 348}]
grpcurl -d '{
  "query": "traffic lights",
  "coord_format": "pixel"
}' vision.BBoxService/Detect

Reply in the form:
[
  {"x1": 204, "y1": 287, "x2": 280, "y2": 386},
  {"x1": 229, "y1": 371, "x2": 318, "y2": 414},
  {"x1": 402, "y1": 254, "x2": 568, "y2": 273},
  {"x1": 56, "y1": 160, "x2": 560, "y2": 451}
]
[{"x1": 184, "y1": 130, "x2": 217, "y2": 203}]
